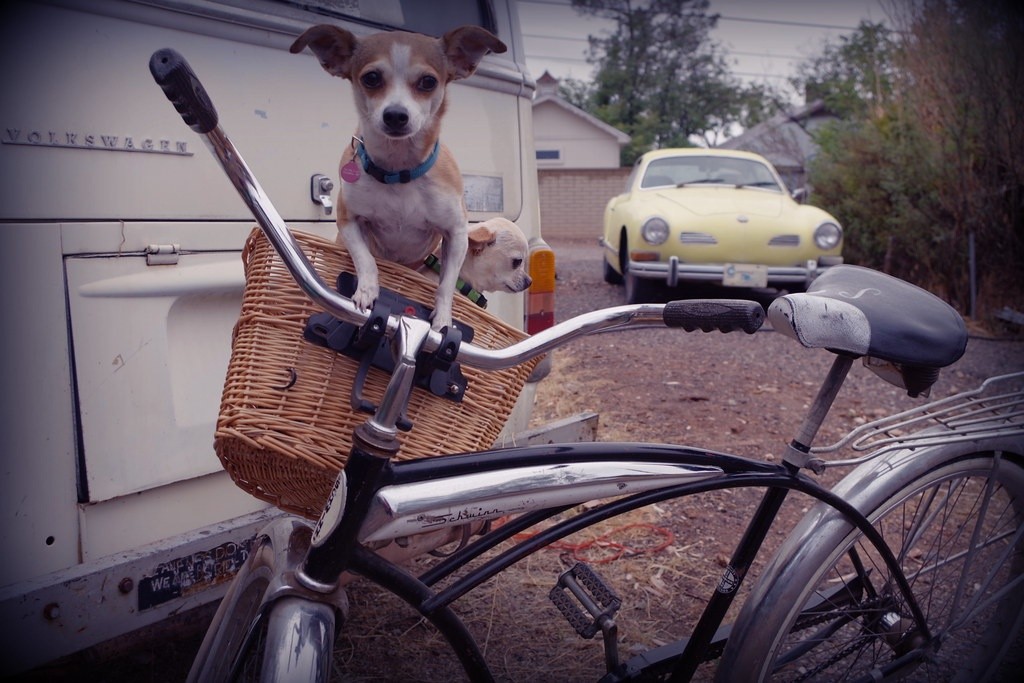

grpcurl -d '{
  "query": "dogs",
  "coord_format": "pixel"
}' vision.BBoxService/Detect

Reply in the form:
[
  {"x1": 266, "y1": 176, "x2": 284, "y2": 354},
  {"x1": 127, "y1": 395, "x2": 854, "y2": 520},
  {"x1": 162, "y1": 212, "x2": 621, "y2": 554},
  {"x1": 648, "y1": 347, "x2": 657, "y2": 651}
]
[{"x1": 289, "y1": 20, "x2": 533, "y2": 333}]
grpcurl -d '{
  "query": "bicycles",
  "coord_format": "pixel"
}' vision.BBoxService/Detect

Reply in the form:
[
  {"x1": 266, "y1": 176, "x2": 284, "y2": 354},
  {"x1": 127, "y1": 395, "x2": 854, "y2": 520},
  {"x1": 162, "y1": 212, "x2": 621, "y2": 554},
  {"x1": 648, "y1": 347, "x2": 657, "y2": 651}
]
[{"x1": 149, "y1": 45, "x2": 1024, "y2": 682}]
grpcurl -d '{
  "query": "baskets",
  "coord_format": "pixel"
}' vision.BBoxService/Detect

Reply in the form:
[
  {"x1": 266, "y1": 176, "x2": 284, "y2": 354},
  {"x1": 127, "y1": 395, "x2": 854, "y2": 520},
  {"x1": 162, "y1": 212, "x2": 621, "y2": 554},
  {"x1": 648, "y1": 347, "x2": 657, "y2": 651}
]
[{"x1": 213, "y1": 223, "x2": 549, "y2": 521}]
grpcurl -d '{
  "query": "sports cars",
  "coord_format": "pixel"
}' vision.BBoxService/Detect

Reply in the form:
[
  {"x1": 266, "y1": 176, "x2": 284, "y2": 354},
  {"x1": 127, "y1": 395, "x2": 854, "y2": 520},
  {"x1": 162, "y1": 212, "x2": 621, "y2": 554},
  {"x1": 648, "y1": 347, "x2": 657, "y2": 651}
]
[{"x1": 597, "y1": 147, "x2": 846, "y2": 304}]
